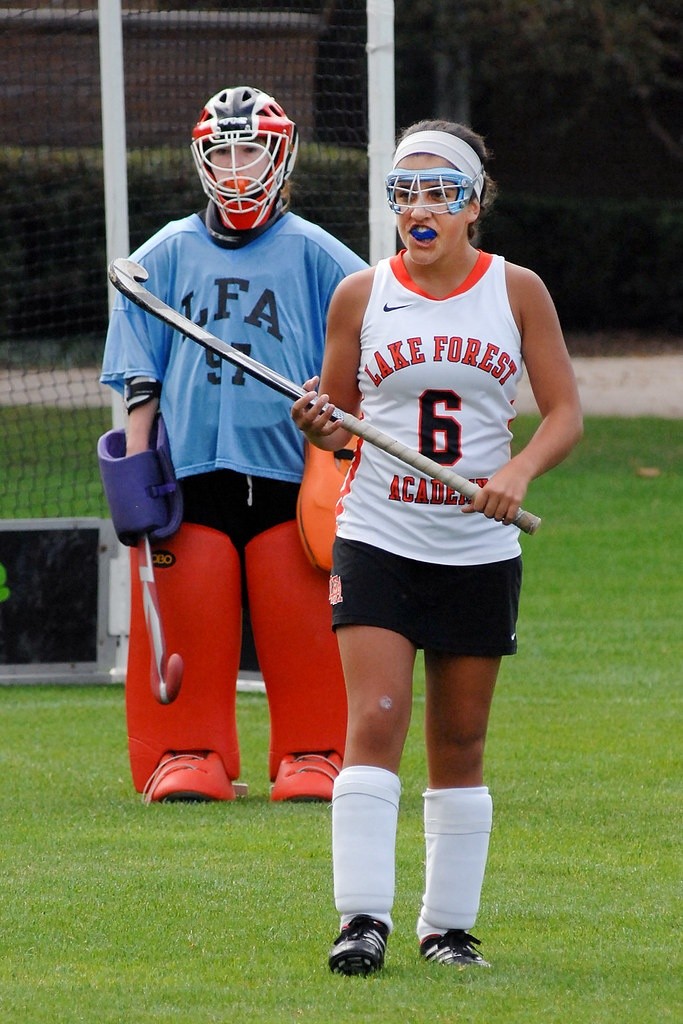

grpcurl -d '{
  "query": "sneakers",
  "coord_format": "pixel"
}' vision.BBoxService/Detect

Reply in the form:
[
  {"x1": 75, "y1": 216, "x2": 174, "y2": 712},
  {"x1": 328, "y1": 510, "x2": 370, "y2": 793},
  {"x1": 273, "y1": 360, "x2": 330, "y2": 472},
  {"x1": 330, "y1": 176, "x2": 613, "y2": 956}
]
[
  {"x1": 419, "y1": 927, "x2": 492, "y2": 970},
  {"x1": 327, "y1": 913, "x2": 389, "y2": 973}
]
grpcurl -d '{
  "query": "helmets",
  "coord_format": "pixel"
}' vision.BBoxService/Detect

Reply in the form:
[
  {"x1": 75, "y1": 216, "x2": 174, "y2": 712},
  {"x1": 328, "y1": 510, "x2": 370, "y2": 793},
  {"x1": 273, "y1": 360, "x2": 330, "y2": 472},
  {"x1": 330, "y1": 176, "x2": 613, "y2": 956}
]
[{"x1": 191, "y1": 85, "x2": 298, "y2": 230}]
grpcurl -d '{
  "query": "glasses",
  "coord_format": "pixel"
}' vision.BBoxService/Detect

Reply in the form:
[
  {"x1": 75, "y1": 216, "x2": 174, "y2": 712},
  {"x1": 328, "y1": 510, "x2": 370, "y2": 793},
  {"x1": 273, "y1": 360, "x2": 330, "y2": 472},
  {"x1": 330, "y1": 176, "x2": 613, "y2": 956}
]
[{"x1": 383, "y1": 166, "x2": 474, "y2": 214}]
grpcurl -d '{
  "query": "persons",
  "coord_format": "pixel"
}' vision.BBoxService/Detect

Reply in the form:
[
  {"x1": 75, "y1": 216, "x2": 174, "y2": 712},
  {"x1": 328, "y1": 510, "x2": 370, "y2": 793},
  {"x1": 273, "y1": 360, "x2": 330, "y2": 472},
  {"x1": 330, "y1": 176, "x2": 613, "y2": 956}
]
[
  {"x1": 288, "y1": 117, "x2": 587, "y2": 985},
  {"x1": 101, "y1": 84, "x2": 371, "y2": 807}
]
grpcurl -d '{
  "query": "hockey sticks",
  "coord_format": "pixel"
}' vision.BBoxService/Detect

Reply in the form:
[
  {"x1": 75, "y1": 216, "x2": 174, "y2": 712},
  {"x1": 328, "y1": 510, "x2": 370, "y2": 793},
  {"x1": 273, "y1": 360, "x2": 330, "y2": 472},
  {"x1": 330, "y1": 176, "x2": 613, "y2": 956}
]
[
  {"x1": 138, "y1": 534, "x2": 186, "y2": 706},
  {"x1": 109, "y1": 257, "x2": 544, "y2": 536}
]
